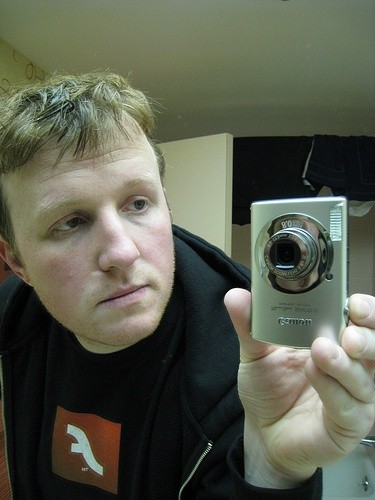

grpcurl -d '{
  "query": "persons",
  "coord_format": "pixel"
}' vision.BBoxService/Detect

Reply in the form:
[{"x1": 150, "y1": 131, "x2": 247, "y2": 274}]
[{"x1": 0, "y1": 68, "x2": 375, "y2": 500}]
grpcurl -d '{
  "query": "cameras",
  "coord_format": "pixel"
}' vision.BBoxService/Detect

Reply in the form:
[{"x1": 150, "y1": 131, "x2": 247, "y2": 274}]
[{"x1": 249, "y1": 196, "x2": 350, "y2": 350}]
